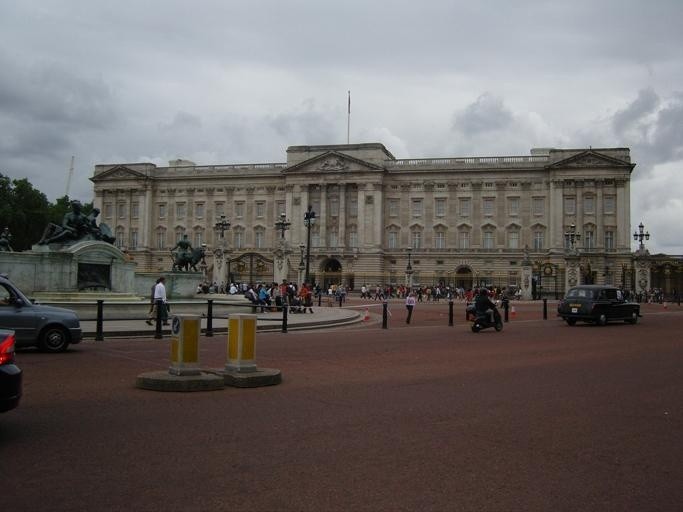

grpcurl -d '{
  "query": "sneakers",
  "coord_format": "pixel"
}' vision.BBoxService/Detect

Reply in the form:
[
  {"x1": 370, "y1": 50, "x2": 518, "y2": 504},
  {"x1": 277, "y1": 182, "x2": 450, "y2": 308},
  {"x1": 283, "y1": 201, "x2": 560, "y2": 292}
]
[
  {"x1": 145, "y1": 320, "x2": 152, "y2": 325},
  {"x1": 162, "y1": 322, "x2": 170, "y2": 326}
]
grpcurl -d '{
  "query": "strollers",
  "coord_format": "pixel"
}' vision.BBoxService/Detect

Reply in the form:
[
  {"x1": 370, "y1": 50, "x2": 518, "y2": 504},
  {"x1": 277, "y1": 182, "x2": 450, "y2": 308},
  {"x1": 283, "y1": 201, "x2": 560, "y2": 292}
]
[{"x1": 288, "y1": 295, "x2": 305, "y2": 313}]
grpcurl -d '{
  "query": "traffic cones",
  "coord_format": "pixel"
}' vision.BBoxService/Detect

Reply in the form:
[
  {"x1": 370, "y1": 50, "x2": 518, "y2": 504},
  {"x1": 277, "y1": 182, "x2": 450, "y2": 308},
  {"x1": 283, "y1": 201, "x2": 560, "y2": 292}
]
[
  {"x1": 662, "y1": 300, "x2": 667, "y2": 311},
  {"x1": 509, "y1": 304, "x2": 516, "y2": 318}
]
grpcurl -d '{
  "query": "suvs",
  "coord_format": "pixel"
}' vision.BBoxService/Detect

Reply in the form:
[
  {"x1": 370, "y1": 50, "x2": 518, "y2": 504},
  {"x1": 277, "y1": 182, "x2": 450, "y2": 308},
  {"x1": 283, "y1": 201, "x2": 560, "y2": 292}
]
[
  {"x1": 0, "y1": 274, "x2": 84, "y2": 352},
  {"x1": 556, "y1": 285, "x2": 641, "y2": 324}
]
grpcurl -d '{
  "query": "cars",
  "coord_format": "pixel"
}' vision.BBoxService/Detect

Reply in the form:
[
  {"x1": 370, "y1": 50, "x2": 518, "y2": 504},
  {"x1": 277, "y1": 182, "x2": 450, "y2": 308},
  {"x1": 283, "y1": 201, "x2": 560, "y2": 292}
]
[{"x1": 0, "y1": 327, "x2": 22, "y2": 411}]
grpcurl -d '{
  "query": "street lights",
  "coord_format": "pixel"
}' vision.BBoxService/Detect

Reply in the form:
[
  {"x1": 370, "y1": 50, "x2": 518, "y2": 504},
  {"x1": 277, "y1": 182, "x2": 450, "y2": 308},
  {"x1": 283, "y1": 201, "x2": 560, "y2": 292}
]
[
  {"x1": 302, "y1": 205, "x2": 316, "y2": 287},
  {"x1": 562, "y1": 222, "x2": 582, "y2": 247},
  {"x1": 215, "y1": 213, "x2": 231, "y2": 239},
  {"x1": 402, "y1": 245, "x2": 415, "y2": 288},
  {"x1": 297, "y1": 241, "x2": 304, "y2": 270},
  {"x1": 274, "y1": 213, "x2": 291, "y2": 240},
  {"x1": 200, "y1": 243, "x2": 208, "y2": 266},
  {"x1": 632, "y1": 220, "x2": 651, "y2": 250}
]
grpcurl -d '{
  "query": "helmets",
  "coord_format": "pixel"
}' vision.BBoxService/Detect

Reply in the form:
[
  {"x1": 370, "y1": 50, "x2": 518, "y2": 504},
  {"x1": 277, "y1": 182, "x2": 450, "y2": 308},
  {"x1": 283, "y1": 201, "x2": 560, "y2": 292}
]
[{"x1": 479, "y1": 287, "x2": 487, "y2": 295}]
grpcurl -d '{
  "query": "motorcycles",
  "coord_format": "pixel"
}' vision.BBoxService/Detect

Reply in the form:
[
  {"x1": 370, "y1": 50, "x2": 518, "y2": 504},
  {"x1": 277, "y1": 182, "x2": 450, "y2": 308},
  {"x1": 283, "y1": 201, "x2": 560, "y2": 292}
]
[{"x1": 467, "y1": 302, "x2": 503, "y2": 333}]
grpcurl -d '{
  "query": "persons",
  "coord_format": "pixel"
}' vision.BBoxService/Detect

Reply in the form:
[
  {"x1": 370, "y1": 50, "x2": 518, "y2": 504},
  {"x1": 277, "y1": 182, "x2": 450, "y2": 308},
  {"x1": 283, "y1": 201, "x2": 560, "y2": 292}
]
[
  {"x1": 617, "y1": 285, "x2": 677, "y2": 304},
  {"x1": 148, "y1": 279, "x2": 160, "y2": 322},
  {"x1": 86, "y1": 208, "x2": 105, "y2": 237},
  {"x1": 146, "y1": 277, "x2": 170, "y2": 325},
  {"x1": 1, "y1": 227, "x2": 13, "y2": 252},
  {"x1": 38, "y1": 200, "x2": 86, "y2": 245},
  {"x1": 170, "y1": 235, "x2": 193, "y2": 271}
]
